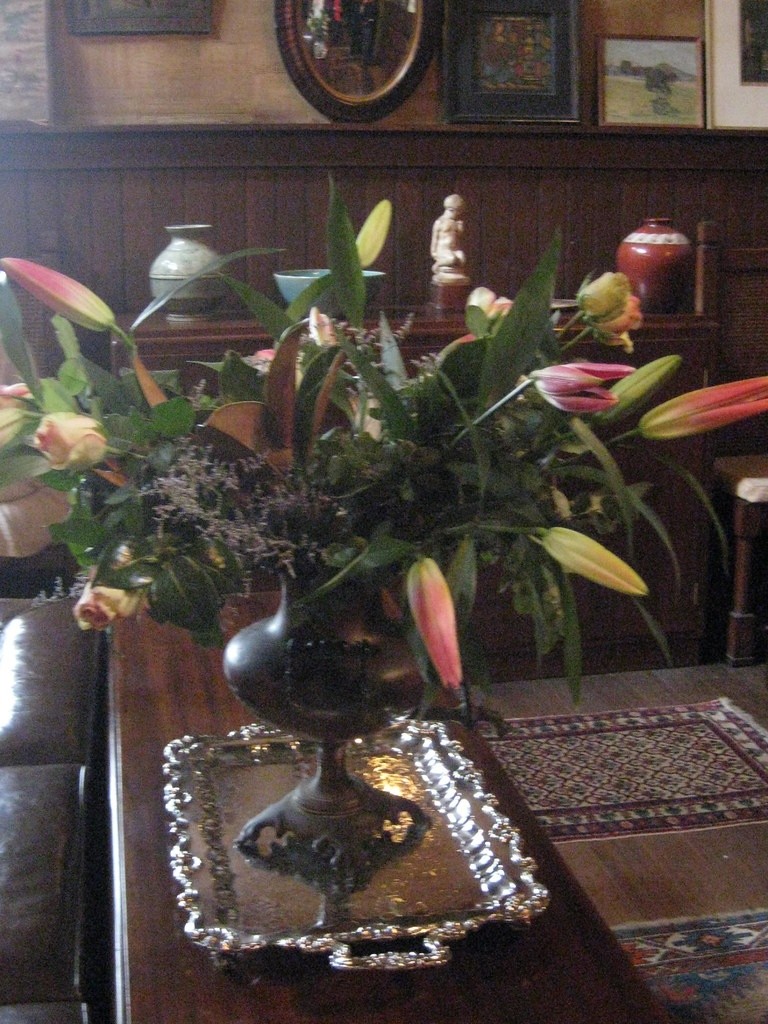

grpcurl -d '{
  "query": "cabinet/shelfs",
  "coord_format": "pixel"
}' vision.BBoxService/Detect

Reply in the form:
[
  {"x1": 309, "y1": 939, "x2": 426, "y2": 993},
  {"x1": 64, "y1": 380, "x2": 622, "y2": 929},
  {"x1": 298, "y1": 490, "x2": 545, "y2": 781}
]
[{"x1": 111, "y1": 306, "x2": 717, "y2": 681}]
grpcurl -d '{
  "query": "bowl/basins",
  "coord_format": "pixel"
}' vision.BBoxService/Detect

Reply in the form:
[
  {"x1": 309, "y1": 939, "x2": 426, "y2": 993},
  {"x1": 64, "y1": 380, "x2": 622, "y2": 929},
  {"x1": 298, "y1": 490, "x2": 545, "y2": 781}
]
[{"x1": 273, "y1": 269, "x2": 387, "y2": 310}]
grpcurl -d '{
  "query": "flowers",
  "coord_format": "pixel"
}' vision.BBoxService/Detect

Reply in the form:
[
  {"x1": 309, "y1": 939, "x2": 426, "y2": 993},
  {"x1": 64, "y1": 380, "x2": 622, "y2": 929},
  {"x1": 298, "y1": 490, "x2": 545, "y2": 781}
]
[{"x1": 1, "y1": 170, "x2": 768, "y2": 728}]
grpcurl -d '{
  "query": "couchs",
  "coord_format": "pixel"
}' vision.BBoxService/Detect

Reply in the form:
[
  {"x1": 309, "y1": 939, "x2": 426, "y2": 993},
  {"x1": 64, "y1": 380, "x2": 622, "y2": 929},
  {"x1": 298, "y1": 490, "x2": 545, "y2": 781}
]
[{"x1": 0, "y1": 588, "x2": 110, "y2": 1024}]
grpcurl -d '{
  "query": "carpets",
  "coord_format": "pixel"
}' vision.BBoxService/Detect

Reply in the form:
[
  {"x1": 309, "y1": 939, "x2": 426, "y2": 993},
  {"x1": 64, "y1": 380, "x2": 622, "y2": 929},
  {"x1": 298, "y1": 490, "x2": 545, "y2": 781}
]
[
  {"x1": 476, "y1": 696, "x2": 768, "y2": 844},
  {"x1": 608, "y1": 907, "x2": 768, "y2": 1024}
]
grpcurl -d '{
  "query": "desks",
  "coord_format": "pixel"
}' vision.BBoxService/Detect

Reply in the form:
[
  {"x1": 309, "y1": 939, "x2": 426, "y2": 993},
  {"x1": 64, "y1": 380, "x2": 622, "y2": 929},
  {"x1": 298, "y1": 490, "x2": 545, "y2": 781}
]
[{"x1": 108, "y1": 592, "x2": 680, "y2": 1024}]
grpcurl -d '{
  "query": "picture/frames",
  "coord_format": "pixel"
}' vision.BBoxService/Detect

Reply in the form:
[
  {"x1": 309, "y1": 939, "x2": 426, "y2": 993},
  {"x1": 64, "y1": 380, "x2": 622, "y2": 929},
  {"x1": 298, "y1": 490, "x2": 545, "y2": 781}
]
[
  {"x1": 64, "y1": 0, "x2": 212, "y2": 33},
  {"x1": 441, "y1": 0, "x2": 582, "y2": 124},
  {"x1": 274, "y1": 0, "x2": 442, "y2": 123},
  {"x1": 596, "y1": 33, "x2": 705, "y2": 128},
  {"x1": 704, "y1": 0, "x2": 768, "y2": 129}
]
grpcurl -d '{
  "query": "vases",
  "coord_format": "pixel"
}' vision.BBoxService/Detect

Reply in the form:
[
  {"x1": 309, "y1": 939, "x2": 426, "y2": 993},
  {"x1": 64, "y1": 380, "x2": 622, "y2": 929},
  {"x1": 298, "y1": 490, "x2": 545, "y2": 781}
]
[
  {"x1": 148, "y1": 225, "x2": 226, "y2": 318},
  {"x1": 223, "y1": 561, "x2": 432, "y2": 893},
  {"x1": 616, "y1": 218, "x2": 693, "y2": 313}
]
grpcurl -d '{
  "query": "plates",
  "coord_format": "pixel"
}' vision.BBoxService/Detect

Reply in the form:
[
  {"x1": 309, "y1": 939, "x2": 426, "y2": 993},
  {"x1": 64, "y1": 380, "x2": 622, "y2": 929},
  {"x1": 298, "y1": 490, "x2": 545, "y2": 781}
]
[{"x1": 549, "y1": 299, "x2": 578, "y2": 310}]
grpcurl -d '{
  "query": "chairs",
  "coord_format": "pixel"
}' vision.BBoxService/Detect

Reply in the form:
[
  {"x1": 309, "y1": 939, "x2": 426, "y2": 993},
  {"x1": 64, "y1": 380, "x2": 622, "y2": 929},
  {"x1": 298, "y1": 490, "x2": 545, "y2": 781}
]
[{"x1": 696, "y1": 221, "x2": 768, "y2": 667}]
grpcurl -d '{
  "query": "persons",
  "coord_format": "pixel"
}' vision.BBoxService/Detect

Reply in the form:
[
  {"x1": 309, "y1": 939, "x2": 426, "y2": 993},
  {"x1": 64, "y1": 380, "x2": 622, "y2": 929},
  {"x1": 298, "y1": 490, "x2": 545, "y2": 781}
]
[
  {"x1": 307, "y1": 0, "x2": 375, "y2": 57},
  {"x1": 431, "y1": 194, "x2": 467, "y2": 273}
]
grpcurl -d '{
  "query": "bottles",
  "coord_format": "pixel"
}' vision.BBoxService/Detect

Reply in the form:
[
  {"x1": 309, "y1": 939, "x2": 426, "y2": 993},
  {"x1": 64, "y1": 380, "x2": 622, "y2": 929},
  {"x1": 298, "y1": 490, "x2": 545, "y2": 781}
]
[{"x1": 618, "y1": 219, "x2": 696, "y2": 313}]
